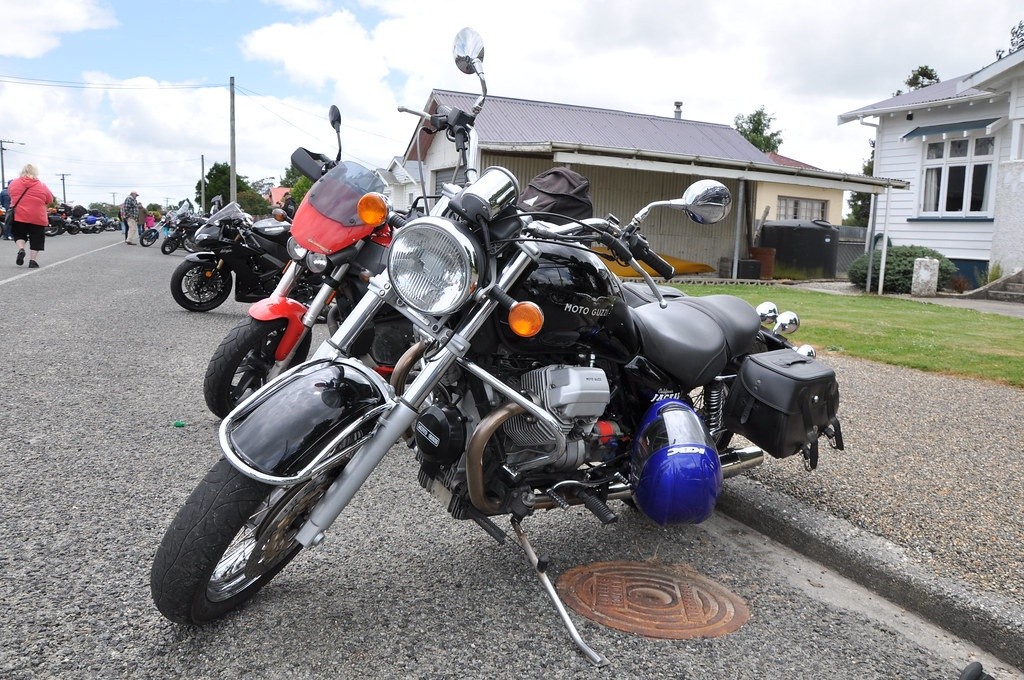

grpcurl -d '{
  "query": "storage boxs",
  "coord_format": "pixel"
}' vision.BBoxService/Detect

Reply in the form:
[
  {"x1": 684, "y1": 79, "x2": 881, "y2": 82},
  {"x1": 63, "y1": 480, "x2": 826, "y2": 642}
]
[{"x1": 719, "y1": 256, "x2": 762, "y2": 278}]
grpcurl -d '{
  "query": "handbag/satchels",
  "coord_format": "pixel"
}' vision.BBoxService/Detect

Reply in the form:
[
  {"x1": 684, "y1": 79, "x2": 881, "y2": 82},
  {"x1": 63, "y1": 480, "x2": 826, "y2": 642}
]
[{"x1": 5, "y1": 206, "x2": 15, "y2": 226}]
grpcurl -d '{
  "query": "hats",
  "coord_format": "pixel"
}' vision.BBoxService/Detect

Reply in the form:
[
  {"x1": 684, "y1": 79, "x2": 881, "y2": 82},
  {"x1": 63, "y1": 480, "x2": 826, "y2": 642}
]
[
  {"x1": 284, "y1": 192, "x2": 291, "y2": 197},
  {"x1": 131, "y1": 191, "x2": 140, "y2": 196}
]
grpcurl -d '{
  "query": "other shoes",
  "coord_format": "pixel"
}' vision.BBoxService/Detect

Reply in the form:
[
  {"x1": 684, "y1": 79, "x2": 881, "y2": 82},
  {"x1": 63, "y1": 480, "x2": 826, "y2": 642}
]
[
  {"x1": 8, "y1": 236, "x2": 13, "y2": 240},
  {"x1": 3, "y1": 236, "x2": 8, "y2": 240}
]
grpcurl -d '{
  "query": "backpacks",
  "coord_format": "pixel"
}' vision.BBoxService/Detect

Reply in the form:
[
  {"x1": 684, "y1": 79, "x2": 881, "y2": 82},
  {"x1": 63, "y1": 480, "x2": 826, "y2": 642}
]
[{"x1": 519, "y1": 166, "x2": 592, "y2": 249}]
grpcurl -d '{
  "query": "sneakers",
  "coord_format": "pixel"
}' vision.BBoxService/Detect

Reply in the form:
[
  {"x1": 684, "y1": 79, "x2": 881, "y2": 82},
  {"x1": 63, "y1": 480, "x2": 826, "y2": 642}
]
[
  {"x1": 29, "y1": 260, "x2": 39, "y2": 268},
  {"x1": 16, "y1": 248, "x2": 26, "y2": 265}
]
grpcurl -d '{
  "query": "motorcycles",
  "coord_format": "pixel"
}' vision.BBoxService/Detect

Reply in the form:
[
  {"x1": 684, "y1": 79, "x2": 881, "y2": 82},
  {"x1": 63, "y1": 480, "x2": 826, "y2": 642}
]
[
  {"x1": 139, "y1": 193, "x2": 255, "y2": 256},
  {"x1": 146, "y1": 28, "x2": 845, "y2": 626},
  {"x1": 202, "y1": 104, "x2": 413, "y2": 424},
  {"x1": 169, "y1": 202, "x2": 294, "y2": 313},
  {"x1": 0, "y1": 201, "x2": 123, "y2": 236}
]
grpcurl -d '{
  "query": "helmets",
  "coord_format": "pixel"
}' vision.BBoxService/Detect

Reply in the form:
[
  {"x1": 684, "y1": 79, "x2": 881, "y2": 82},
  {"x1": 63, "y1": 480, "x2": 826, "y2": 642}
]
[{"x1": 630, "y1": 399, "x2": 722, "y2": 526}]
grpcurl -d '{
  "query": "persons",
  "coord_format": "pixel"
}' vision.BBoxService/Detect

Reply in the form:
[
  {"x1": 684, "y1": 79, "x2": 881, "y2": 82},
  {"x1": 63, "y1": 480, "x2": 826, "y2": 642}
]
[
  {"x1": 282, "y1": 192, "x2": 298, "y2": 218},
  {"x1": 145, "y1": 211, "x2": 155, "y2": 229},
  {"x1": 162, "y1": 214, "x2": 171, "y2": 239},
  {"x1": 8, "y1": 163, "x2": 52, "y2": 268},
  {"x1": 124, "y1": 191, "x2": 141, "y2": 245},
  {"x1": 118, "y1": 204, "x2": 129, "y2": 240},
  {"x1": 137, "y1": 202, "x2": 154, "y2": 238},
  {"x1": 0, "y1": 179, "x2": 13, "y2": 240}
]
